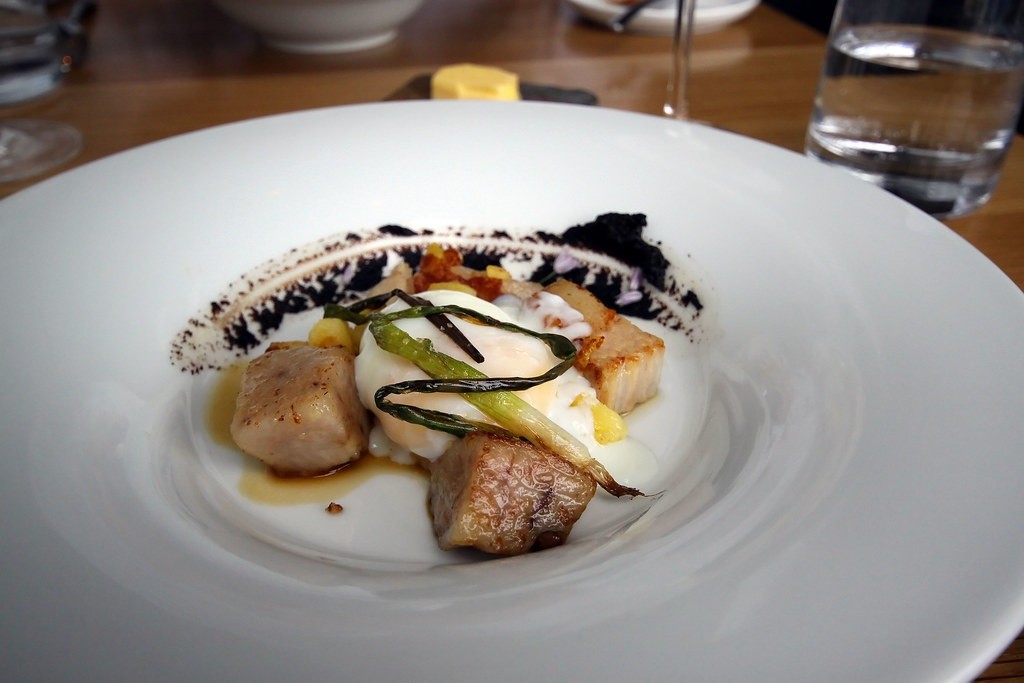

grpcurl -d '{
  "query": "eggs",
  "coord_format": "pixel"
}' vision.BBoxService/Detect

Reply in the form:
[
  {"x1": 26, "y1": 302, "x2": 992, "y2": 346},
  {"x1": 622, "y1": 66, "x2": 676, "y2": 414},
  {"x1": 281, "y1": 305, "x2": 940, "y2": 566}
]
[{"x1": 357, "y1": 288, "x2": 626, "y2": 464}]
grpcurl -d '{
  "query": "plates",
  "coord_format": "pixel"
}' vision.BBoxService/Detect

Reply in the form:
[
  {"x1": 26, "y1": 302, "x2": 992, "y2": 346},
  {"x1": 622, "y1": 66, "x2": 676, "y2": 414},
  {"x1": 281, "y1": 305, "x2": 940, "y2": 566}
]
[
  {"x1": 0, "y1": 100, "x2": 1024, "y2": 682},
  {"x1": 569, "y1": 0, "x2": 760, "y2": 35}
]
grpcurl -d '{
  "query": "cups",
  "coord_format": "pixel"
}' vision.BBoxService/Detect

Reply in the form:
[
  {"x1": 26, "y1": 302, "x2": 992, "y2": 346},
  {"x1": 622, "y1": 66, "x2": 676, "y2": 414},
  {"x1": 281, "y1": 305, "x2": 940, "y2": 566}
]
[{"x1": 803, "y1": 0, "x2": 1024, "y2": 219}]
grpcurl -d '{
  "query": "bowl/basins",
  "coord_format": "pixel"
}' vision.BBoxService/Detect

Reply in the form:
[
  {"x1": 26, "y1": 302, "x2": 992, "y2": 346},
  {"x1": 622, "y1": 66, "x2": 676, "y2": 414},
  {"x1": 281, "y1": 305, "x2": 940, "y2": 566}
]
[{"x1": 219, "y1": 0, "x2": 422, "y2": 56}]
